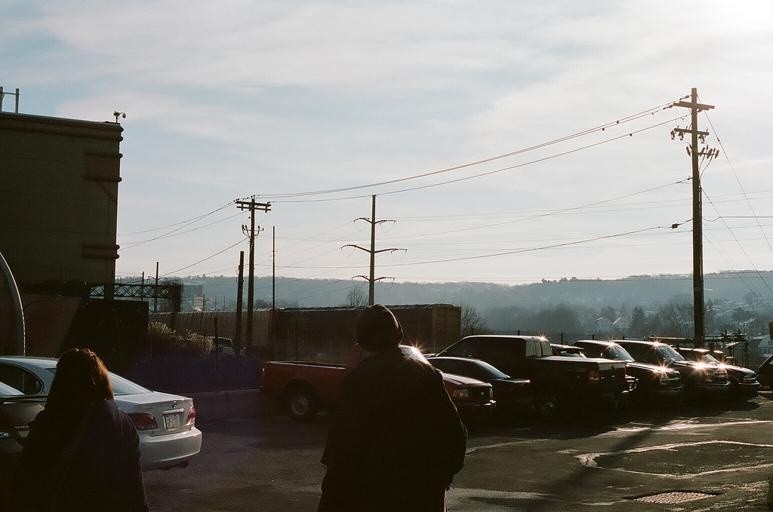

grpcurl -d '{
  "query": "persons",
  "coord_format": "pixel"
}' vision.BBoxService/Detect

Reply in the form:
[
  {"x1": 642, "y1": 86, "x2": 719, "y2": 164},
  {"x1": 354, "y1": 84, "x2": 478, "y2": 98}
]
[
  {"x1": 9, "y1": 347, "x2": 151, "y2": 511},
  {"x1": 316, "y1": 302, "x2": 466, "y2": 511}
]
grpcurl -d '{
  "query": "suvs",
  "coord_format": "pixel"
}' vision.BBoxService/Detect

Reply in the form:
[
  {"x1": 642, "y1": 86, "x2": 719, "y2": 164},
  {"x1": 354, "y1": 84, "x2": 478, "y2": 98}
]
[
  {"x1": 608, "y1": 338, "x2": 731, "y2": 402},
  {"x1": 202, "y1": 336, "x2": 235, "y2": 359}
]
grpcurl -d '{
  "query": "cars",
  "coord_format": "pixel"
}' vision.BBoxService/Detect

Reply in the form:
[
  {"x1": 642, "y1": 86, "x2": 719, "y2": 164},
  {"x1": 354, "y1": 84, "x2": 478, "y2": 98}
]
[
  {"x1": 0, "y1": 356, "x2": 204, "y2": 471},
  {"x1": 671, "y1": 348, "x2": 761, "y2": 402},
  {"x1": 0, "y1": 381, "x2": 51, "y2": 461},
  {"x1": 428, "y1": 357, "x2": 535, "y2": 412},
  {"x1": 550, "y1": 344, "x2": 640, "y2": 395},
  {"x1": 572, "y1": 340, "x2": 683, "y2": 404}
]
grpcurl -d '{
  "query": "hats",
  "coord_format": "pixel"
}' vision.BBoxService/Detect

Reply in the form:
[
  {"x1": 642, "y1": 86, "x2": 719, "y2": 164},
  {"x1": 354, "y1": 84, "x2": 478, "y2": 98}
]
[{"x1": 357, "y1": 303, "x2": 404, "y2": 353}]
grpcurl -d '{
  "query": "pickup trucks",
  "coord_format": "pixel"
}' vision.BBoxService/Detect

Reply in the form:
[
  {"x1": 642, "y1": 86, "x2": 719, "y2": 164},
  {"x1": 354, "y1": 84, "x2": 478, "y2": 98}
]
[
  {"x1": 259, "y1": 345, "x2": 497, "y2": 421},
  {"x1": 423, "y1": 334, "x2": 626, "y2": 423}
]
